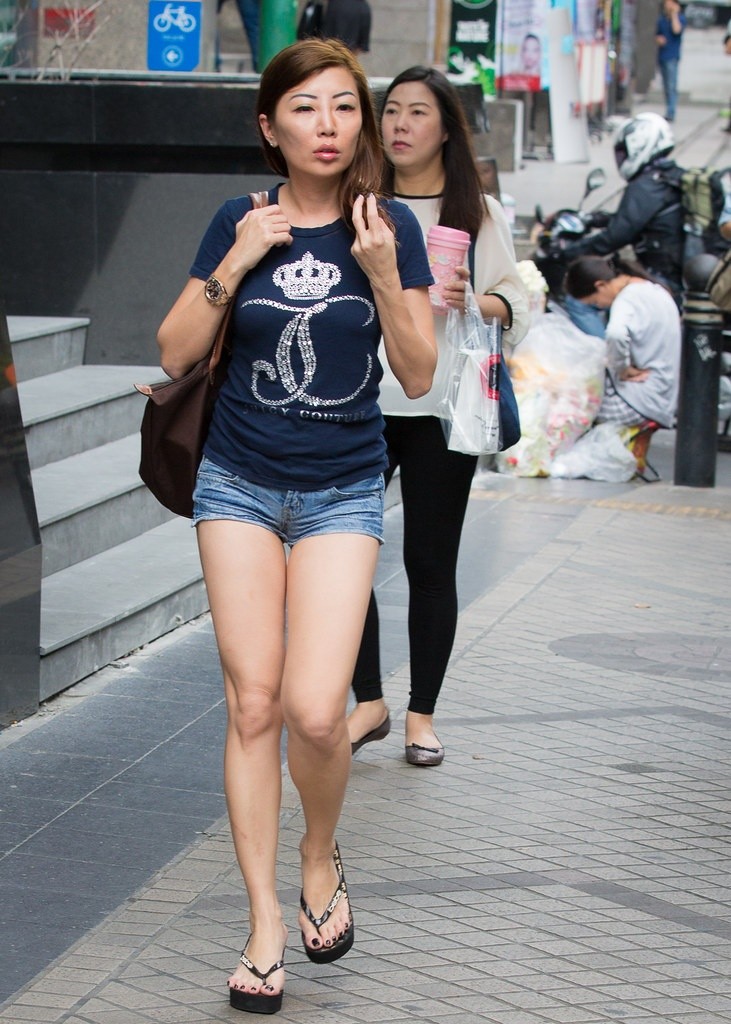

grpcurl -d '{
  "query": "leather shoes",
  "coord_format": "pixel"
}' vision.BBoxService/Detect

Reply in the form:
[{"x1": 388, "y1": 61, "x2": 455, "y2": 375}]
[
  {"x1": 406, "y1": 723, "x2": 445, "y2": 765},
  {"x1": 351, "y1": 716, "x2": 390, "y2": 755}
]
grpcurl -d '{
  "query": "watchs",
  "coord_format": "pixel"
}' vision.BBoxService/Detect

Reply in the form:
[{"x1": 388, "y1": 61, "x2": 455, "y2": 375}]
[{"x1": 204, "y1": 274, "x2": 234, "y2": 306}]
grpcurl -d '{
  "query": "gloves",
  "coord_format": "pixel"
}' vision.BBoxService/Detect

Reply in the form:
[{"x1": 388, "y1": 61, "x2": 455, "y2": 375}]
[
  {"x1": 564, "y1": 246, "x2": 594, "y2": 262},
  {"x1": 591, "y1": 210, "x2": 614, "y2": 228}
]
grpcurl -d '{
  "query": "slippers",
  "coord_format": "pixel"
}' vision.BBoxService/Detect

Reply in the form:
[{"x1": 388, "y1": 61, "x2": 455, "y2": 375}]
[
  {"x1": 230, "y1": 933, "x2": 283, "y2": 1013},
  {"x1": 300, "y1": 837, "x2": 354, "y2": 965}
]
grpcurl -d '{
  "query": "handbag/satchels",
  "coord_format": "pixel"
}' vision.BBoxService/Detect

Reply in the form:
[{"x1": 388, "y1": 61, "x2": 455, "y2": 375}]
[
  {"x1": 435, "y1": 283, "x2": 503, "y2": 454},
  {"x1": 463, "y1": 193, "x2": 521, "y2": 451},
  {"x1": 134, "y1": 191, "x2": 269, "y2": 518}
]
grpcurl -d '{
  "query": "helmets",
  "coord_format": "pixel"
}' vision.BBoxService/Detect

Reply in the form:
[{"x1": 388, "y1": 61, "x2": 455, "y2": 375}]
[{"x1": 614, "y1": 111, "x2": 676, "y2": 181}]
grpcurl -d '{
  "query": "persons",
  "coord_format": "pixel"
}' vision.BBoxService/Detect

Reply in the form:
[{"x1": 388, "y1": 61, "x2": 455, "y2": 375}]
[
  {"x1": 215, "y1": 0, "x2": 259, "y2": 72},
  {"x1": 296, "y1": 0, "x2": 373, "y2": 54},
  {"x1": 654, "y1": 0, "x2": 686, "y2": 120},
  {"x1": 554, "y1": 111, "x2": 686, "y2": 340},
  {"x1": 345, "y1": 66, "x2": 529, "y2": 766},
  {"x1": 566, "y1": 253, "x2": 683, "y2": 429},
  {"x1": 154, "y1": 36, "x2": 439, "y2": 1016},
  {"x1": 517, "y1": 34, "x2": 540, "y2": 79},
  {"x1": 685, "y1": 166, "x2": 731, "y2": 313}
]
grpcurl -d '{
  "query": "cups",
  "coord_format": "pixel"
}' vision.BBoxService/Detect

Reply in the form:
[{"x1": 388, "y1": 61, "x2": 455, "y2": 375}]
[{"x1": 422, "y1": 226, "x2": 471, "y2": 316}]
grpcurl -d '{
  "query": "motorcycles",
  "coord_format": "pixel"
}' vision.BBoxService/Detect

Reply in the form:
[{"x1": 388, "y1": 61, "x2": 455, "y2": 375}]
[{"x1": 524, "y1": 168, "x2": 731, "y2": 438}]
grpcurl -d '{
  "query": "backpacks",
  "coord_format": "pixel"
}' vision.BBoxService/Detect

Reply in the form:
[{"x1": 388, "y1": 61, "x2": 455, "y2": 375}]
[{"x1": 660, "y1": 167, "x2": 726, "y2": 238}]
